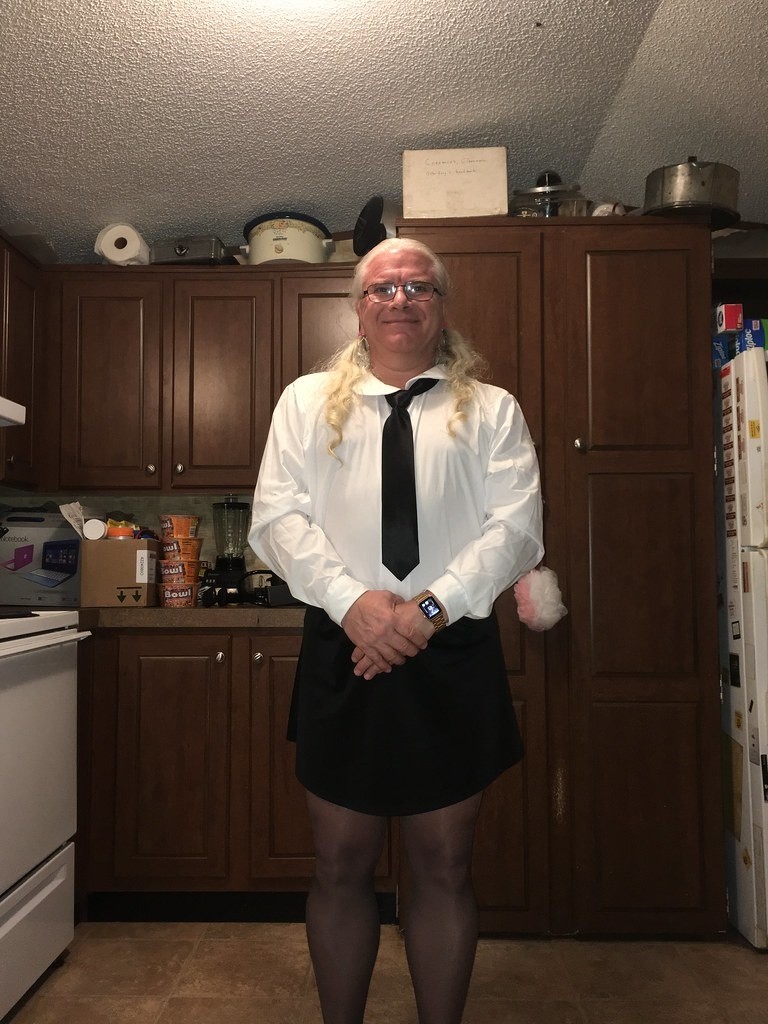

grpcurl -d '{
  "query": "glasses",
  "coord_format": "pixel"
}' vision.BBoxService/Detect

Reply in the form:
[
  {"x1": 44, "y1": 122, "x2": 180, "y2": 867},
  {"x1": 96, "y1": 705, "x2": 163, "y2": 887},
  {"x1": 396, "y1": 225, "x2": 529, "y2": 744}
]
[{"x1": 364, "y1": 280, "x2": 443, "y2": 303}]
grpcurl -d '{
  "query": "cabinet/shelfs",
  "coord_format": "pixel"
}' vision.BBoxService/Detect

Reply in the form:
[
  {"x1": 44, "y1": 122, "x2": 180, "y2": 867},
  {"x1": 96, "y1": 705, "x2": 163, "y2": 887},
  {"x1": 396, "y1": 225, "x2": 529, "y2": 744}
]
[{"x1": 0, "y1": 213, "x2": 727, "y2": 922}]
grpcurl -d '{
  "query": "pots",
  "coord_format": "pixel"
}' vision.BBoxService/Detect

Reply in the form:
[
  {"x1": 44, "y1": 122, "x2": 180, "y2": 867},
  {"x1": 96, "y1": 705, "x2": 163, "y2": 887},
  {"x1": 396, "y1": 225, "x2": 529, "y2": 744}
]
[{"x1": 642, "y1": 156, "x2": 741, "y2": 221}]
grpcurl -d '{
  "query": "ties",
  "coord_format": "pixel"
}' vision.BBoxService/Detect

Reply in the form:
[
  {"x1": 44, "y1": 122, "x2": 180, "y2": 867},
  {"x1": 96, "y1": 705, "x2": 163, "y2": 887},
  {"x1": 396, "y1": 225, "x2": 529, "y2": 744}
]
[{"x1": 382, "y1": 378, "x2": 439, "y2": 582}]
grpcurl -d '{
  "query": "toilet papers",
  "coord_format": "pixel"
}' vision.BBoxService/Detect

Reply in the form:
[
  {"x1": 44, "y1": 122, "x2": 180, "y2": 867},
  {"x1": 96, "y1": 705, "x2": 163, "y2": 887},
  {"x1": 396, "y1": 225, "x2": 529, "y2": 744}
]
[{"x1": 94, "y1": 221, "x2": 148, "y2": 267}]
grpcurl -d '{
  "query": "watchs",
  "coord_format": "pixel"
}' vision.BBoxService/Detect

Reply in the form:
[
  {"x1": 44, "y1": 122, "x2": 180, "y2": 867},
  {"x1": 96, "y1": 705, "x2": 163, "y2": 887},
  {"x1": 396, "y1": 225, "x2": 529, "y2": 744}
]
[{"x1": 412, "y1": 592, "x2": 447, "y2": 635}]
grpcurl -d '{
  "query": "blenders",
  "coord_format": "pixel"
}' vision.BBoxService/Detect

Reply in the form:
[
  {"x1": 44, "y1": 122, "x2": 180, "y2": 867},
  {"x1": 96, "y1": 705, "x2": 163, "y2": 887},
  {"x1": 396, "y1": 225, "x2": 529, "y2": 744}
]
[{"x1": 203, "y1": 494, "x2": 250, "y2": 588}]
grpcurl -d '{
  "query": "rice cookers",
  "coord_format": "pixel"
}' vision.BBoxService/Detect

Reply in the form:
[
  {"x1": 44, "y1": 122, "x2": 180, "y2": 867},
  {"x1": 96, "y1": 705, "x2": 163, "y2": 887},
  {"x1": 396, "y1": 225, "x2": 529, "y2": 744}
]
[{"x1": 240, "y1": 212, "x2": 337, "y2": 265}]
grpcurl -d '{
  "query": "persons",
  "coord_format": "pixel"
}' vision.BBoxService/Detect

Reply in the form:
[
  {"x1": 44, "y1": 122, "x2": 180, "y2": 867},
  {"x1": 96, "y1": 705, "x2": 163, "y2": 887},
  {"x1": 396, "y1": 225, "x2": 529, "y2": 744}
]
[
  {"x1": 246, "y1": 238, "x2": 546, "y2": 1024},
  {"x1": 423, "y1": 601, "x2": 439, "y2": 617}
]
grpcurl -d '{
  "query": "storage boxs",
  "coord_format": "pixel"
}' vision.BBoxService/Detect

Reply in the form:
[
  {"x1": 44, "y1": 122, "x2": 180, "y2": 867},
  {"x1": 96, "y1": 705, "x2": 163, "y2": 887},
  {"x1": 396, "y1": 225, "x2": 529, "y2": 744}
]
[
  {"x1": 0, "y1": 508, "x2": 106, "y2": 606},
  {"x1": 403, "y1": 146, "x2": 508, "y2": 218},
  {"x1": 712, "y1": 302, "x2": 763, "y2": 371},
  {"x1": 80, "y1": 538, "x2": 160, "y2": 607}
]
